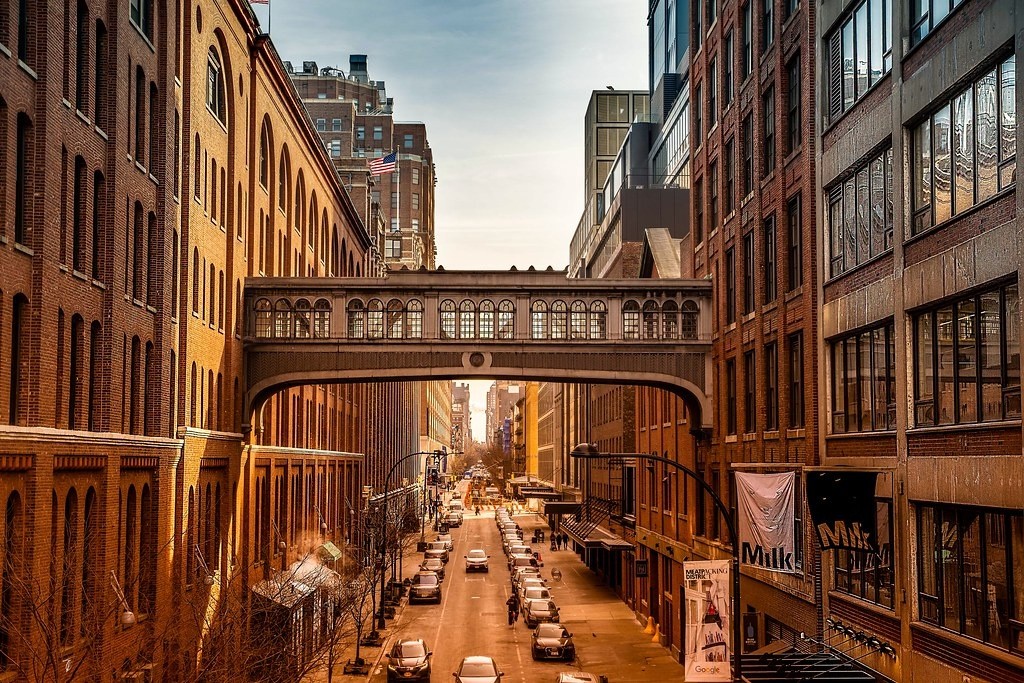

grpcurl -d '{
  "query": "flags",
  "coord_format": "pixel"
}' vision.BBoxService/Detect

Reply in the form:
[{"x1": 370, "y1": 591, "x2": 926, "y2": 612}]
[{"x1": 368, "y1": 150, "x2": 396, "y2": 177}]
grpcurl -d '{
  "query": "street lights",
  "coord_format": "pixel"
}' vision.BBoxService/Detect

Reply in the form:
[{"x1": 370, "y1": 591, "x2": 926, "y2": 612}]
[
  {"x1": 570, "y1": 443, "x2": 742, "y2": 683},
  {"x1": 380, "y1": 449, "x2": 448, "y2": 618}
]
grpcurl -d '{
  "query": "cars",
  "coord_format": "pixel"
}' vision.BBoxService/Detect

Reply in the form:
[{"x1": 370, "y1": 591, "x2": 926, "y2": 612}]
[
  {"x1": 452, "y1": 655, "x2": 505, "y2": 683},
  {"x1": 384, "y1": 638, "x2": 433, "y2": 683},
  {"x1": 463, "y1": 549, "x2": 491, "y2": 573},
  {"x1": 515, "y1": 577, "x2": 552, "y2": 598},
  {"x1": 423, "y1": 542, "x2": 449, "y2": 564},
  {"x1": 494, "y1": 505, "x2": 548, "y2": 593},
  {"x1": 464, "y1": 475, "x2": 471, "y2": 480},
  {"x1": 452, "y1": 491, "x2": 463, "y2": 499},
  {"x1": 434, "y1": 533, "x2": 454, "y2": 552},
  {"x1": 555, "y1": 672, "x2": 599, "y2": 683},
  {"x1": 418, "y1": 558, "x2": 446, "y2": 579},
  {"x1": 523, "y1": 599, "x2": 561, "y2": 629},
  {"x1": 443, "y1": 513, "x2": 462, "y2": 528},
  {"x1": 519, "y1": 586, "x2": 555, "y2": 613},
  {"x1": 530, "y1": 622, "x2": 575, "y2": 662},
  {"x1": 408, "y1": 571, "x2": 442, "y2": 605}
]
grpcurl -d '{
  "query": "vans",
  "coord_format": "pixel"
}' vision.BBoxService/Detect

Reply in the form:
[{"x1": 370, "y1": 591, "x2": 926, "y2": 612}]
[{"x1": 447, "y1": 500, "x2": 464, "y2": 521}]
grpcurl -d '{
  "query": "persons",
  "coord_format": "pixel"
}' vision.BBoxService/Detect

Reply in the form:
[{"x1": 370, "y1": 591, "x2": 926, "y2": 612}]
[
  {"x1": 506, "y1": 594, "x2": 521, "y2": 625},
  {"x1": 427, "y1": 499, "x2": 569, "y2": 552}
]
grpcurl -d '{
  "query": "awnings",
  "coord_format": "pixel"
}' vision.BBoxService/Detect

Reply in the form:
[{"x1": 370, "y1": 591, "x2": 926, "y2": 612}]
[{"x1": 250, "y1": 542, "x2": 346, "y2": 609}]
